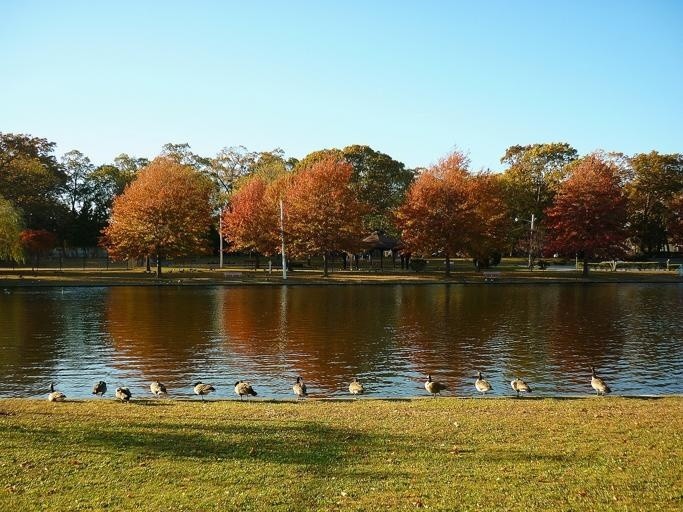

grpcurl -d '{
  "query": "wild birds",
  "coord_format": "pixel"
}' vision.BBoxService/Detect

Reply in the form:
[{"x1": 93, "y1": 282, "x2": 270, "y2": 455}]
[
  {"x1": 589, "y1": 364, "x2": 612, "y2": 396},
  {"x1": 292, "y1": 376, "x2": 308, "y2": 396},
  {"x1": 474, "y1": 371, "x2": 533, "y2": 397},
  {"x1": 193, "y1": 381, "x2": 216, "y2": 399},
  {"x1": 233, "y1": 380, "x2": 258, "y2": 398},
  {"x1": 423, "y1": 374, "x2": 449, "y2": 399},
  {"x1": 47, "y1": 381, "x2": 66, "y2": 402},
  {"x1": 347, "y1": 376, "x2": 366, "y2": 395},
  {"x1": 149, "y1": 379, "x2": 167, "y2": 396},
  {"x1": 91, "y1": 380, "x2": 132, "y2": 402}
]
